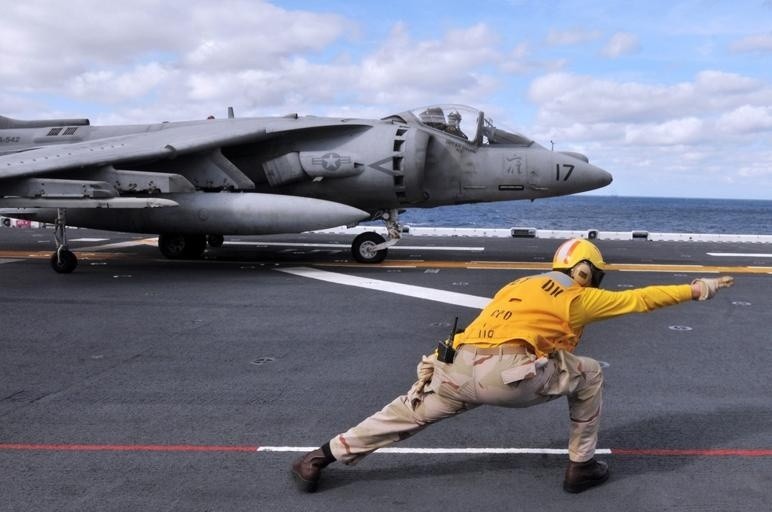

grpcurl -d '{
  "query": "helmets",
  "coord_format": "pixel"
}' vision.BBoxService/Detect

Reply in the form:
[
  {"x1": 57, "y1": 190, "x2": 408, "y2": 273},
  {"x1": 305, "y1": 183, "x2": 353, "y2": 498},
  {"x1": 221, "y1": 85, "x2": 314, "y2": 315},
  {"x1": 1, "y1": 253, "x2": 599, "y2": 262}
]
[{"x1": 552, "y1": 238, "x2": 606, "y2": 270}]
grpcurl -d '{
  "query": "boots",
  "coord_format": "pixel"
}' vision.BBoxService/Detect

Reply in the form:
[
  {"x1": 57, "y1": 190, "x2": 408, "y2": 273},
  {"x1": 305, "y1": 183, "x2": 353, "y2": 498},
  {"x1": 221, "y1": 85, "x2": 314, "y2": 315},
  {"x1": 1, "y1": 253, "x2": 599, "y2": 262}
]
[
  {"x1": 290, "y1": 448, "x2": 331, "y2": 494},
  {"x1": 564, "y1": 458, "x2": 610, "y2": 494}
]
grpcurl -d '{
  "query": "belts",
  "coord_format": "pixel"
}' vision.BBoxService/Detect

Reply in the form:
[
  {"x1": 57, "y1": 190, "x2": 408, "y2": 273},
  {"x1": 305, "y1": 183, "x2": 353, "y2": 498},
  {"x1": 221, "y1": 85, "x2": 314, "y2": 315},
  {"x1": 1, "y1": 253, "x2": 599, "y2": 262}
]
[{"x1": 462, "y1": 346, "x2": 526, "y2": 355}]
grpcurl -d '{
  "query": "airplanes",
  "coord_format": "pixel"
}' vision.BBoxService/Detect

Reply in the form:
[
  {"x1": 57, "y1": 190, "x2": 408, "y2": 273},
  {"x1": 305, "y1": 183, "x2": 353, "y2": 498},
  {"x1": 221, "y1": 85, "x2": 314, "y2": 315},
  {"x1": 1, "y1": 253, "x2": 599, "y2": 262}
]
[{"x1": 0, "y1": 102, "x2": 612, "y2": 275}]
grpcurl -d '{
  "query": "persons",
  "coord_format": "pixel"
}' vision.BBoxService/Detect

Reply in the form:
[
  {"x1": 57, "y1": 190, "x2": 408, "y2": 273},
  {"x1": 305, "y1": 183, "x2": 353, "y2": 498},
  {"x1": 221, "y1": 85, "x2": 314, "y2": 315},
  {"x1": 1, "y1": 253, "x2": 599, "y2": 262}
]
[{"x1": 289, "y1": 237, "x2": 735, "y2": 493}]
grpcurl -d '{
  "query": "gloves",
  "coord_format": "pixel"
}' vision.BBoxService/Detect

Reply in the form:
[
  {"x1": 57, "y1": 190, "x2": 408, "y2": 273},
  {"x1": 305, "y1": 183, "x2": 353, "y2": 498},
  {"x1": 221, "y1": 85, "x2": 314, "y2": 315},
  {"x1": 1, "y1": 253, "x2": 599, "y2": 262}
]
[{"x1": 690, "y1": 276, "x2": 735, "y2": 302}]
